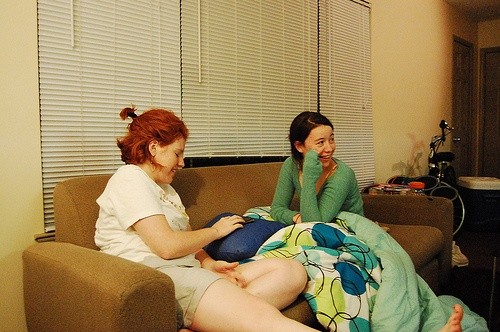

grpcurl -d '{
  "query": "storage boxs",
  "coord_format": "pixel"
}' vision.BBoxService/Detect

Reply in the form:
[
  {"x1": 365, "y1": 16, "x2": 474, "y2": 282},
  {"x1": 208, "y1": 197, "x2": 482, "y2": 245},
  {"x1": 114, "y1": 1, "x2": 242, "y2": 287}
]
[{"x1": 457, "y1": 176, "x2": 500, "y2": 233}]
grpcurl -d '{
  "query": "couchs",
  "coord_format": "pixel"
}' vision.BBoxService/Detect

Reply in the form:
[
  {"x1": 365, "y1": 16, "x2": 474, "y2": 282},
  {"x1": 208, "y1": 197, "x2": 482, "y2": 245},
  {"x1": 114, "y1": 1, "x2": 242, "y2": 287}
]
[{"x1": 21, "y1": 162, "x2": 453, "y2": 332}]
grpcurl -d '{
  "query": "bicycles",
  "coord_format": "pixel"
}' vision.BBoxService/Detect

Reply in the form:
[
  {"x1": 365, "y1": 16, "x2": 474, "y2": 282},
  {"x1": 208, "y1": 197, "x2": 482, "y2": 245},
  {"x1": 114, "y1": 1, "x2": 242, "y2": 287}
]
[{"x1": 428, "y1": 120, "x2": 467, "y2": 239}]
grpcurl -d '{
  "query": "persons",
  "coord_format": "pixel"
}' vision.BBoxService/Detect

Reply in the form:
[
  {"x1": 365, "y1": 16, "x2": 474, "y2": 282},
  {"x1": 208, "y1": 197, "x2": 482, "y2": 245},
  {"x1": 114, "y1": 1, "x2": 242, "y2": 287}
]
[
  {"x1": 267, "y1": 109, "x2": 363, "y2": 227},
  {"x1": 92, "y1": 107, "x2": 464, "y2": 332}
]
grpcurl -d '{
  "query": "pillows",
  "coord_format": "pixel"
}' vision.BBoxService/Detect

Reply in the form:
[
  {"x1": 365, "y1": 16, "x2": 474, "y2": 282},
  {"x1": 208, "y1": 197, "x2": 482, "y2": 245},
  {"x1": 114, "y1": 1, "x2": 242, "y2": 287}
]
[{"x1": 202, "y1": 213, "x2": 288, "y2": 263}]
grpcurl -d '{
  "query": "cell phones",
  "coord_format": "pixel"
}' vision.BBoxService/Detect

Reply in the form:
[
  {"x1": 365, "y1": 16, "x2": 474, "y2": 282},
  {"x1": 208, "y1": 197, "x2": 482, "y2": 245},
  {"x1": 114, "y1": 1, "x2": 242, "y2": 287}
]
[{"x1": 236, "y1": 217, "x2": 253, "y2": 225}]
